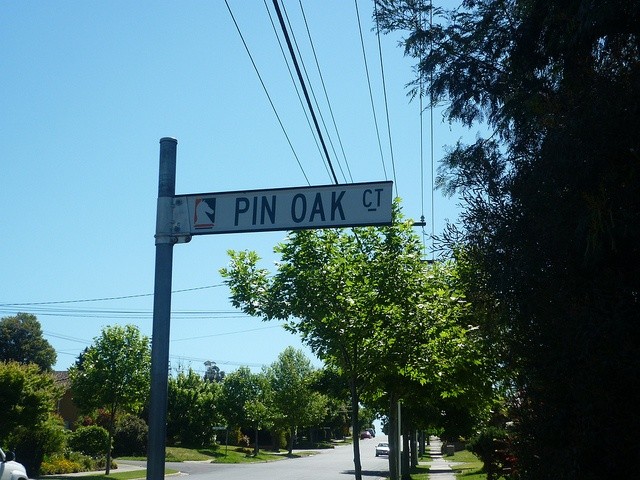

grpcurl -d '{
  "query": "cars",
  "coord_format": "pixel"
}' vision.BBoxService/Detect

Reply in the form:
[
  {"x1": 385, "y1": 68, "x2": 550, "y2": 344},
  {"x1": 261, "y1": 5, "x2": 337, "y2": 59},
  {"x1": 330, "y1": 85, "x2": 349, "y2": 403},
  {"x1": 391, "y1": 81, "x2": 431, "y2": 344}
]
[
  {"x1": 375, "y1": 442, "x2": 389, "y2": 457},
  {"x1": 361, "y1": 431, "x2": 371, "y2": 440},
  {"x1": 0, "y1": 449, "x2": 27, "y2": 479}
]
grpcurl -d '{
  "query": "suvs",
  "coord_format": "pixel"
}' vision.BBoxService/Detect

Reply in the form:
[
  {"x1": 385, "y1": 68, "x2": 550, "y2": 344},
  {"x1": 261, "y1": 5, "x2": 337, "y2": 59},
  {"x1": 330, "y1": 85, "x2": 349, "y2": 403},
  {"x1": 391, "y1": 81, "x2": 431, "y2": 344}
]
[{"x1": 366, "y1": 428, "x2": 375, "y2": 437}]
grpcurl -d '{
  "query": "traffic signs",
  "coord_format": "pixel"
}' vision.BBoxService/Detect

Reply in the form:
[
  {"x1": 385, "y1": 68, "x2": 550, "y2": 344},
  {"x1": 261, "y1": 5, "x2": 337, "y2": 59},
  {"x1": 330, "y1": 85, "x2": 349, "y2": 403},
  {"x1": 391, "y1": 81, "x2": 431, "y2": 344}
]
[{"x1": 157, "y1": 182, "x2": 391, "y2": 242}]
